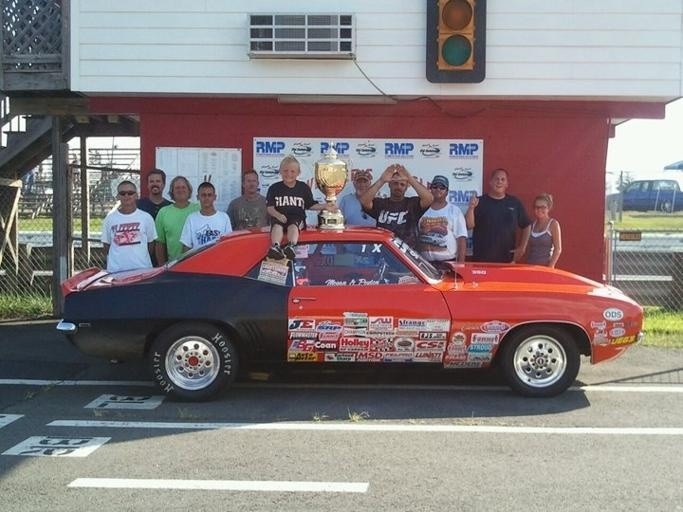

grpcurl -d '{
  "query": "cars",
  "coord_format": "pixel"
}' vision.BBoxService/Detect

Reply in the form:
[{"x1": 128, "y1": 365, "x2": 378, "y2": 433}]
[
  {"x1": 56, "y1": 225, "x2": 644, "y2": 397},
  {"x1": 607, "y1": 180, "x2": 683, "y2": 213}
]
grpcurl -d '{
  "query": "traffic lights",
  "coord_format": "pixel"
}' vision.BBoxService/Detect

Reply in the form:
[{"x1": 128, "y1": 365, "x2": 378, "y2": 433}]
[{"x1": 427, "y1": 0, "x2": 486, "y2": 83}]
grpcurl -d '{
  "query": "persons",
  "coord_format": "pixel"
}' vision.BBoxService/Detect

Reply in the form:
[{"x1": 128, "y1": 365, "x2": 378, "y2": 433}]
[
  {"x1": 266, "y1": 155, "x2": 338, "y2": 260},
  {"x1": 154, "y1": 176, "x2": 201, "y2": 269},
  {"x1": 360, "y1": 164, "x2": 434, "y2": 250},
  {"x1": 465, "y1": 168, "x2": 533, "y2": 263},
  {"x1": 135, "y1": 169, "x2": 174, "y2": 267},
  {"x1": 226, "y1": 168, "x2": 272, "y2": 231},
  {"x1": 100, "y1": 180, "x2": 159, "y2": 270},
  {"x1": 527, "y1": 192, "x2": 562, "y2": 268},
  {"x1": 341, "y1": 169, "x2": 377, "y2": 228},
  {"x1": 176, "y1": 182, "x2": 233, "y2": 258},
  {"x1": 417, "y1": 175, "x2": 468, "y2": 264}
]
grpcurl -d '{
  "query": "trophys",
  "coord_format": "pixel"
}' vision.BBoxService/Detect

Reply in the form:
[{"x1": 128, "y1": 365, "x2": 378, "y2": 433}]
[{"x1": 314, "y1": 140, "x2": 348, "y2": 233}]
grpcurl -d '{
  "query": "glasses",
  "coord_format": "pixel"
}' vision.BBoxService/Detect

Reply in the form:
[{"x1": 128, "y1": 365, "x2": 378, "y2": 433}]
[
  {"x1": 119, "y1": 191, "x2": 136, "y2": 196},
  {"x1": 432, "y1": 185, "x2": 445, "y2": 190}
]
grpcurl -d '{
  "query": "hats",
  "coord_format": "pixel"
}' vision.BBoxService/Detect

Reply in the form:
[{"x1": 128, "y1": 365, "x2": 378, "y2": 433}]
[
  {"x1": 433, "y1": 176, "x2": 449, "y2": 188},
  {"x1": 354, "y1": 172, "x2": 373, "y2": 181}
]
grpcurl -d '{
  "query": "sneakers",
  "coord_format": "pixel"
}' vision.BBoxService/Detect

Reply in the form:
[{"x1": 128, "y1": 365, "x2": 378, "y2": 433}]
[
  {"x1": 268, "y1": 241, "x2": 284, "y2": 259},
  {"x1": 283, "y1": 240, "x2": 297, "y2": 259}
]
